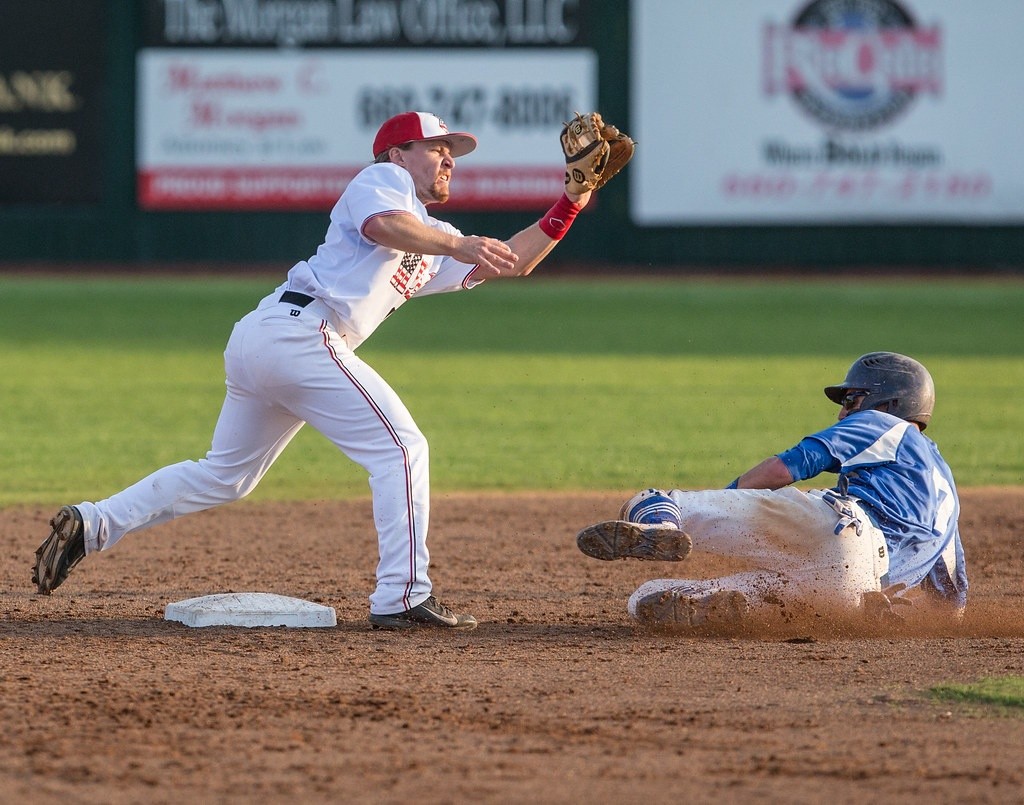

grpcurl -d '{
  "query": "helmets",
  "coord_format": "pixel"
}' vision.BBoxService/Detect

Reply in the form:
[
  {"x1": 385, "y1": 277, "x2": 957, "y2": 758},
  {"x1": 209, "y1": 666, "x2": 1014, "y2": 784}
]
[{"x1": 825, "y1": 351, "x2": 935, "y2": 431}]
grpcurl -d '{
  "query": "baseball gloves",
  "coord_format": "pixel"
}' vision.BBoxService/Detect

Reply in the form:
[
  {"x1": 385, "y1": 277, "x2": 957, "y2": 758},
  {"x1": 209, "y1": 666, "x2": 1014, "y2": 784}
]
[{"x1": 558, "y1": 109, "x2": 641, "y2": 196}]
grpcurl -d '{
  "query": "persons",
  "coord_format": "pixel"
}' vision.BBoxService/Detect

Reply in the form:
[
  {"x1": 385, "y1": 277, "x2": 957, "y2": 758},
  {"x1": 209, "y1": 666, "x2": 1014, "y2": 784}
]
[
  {"x1": 33, "y1": 109, "x2": 633, "y2": 628},
  {"x1": 578, "y1": 353, "x2": 969, "y2": 637}
]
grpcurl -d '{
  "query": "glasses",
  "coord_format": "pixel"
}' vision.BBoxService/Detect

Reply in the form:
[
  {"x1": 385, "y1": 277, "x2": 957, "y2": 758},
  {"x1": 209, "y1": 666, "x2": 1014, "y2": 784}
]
[{"x1": 843, "y1": 392, "x2": 866, "y2": 409}]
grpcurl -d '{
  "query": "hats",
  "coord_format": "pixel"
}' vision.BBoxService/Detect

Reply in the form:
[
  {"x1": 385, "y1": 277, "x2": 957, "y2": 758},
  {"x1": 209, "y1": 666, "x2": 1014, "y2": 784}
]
[{"x1": 373, "y1": 111, "x2": 477, "y2": 157}]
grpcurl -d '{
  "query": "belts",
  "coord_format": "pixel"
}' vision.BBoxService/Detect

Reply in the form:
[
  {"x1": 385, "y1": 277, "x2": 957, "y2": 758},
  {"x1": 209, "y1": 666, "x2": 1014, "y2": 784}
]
[{"x1": 280, "y1": 290, "x2": 315, "y2": 308}]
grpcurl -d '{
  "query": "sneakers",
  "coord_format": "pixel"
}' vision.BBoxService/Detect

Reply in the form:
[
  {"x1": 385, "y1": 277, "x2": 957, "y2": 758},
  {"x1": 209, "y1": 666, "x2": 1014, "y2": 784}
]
[
  {"x1": 31, "y1": 505, "x2": 86, "y2": 590},
  {"x1": 636, "y1": 586, "x2": 749, "y2": 632},
  {"x1": 578, "y1": 521, "x2": 693, "y2": 561},
  {"x1": 368, "y1": 596, "x2": 478, "y2": 631}
]
